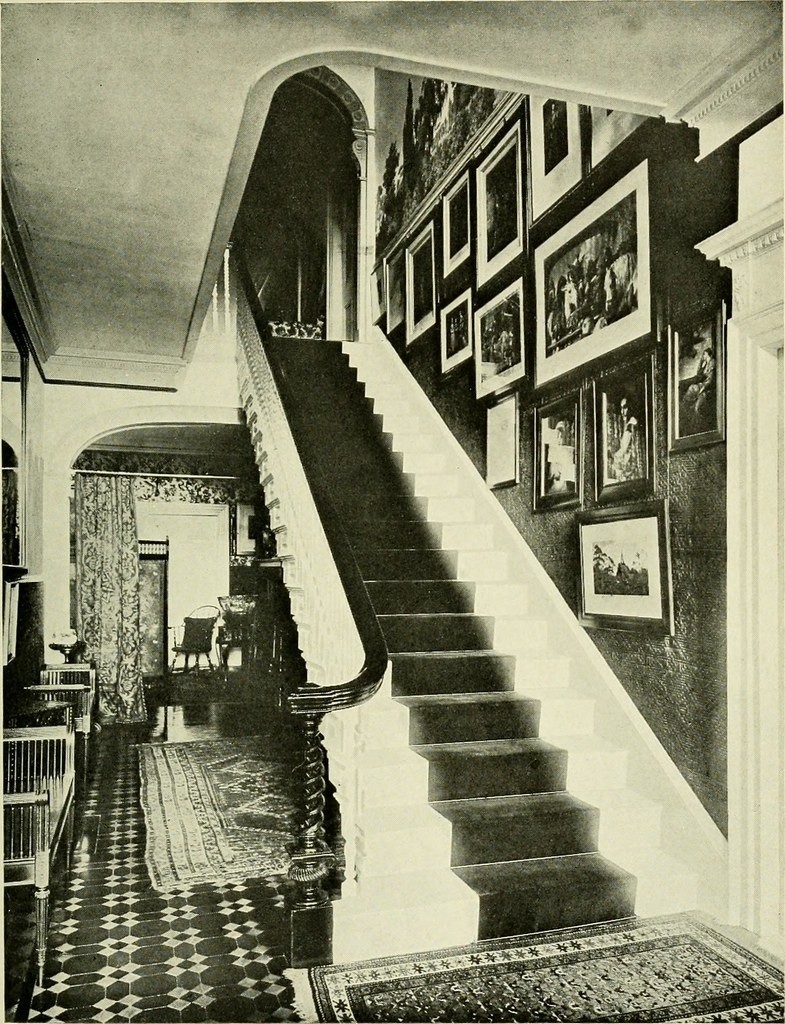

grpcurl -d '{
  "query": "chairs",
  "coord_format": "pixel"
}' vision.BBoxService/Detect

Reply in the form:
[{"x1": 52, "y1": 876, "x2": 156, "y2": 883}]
[{"x1": 166, "y1": 605, "x2": 221, "y2": 676}]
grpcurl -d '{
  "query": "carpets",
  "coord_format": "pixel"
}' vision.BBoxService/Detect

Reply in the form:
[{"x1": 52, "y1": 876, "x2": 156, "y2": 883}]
[
  {"x1": 281, "y1": 910, "x2": 785, "y2": 1024},
  {"x1": 130, "y1": 732, "x2": 347, "y2": 892}
]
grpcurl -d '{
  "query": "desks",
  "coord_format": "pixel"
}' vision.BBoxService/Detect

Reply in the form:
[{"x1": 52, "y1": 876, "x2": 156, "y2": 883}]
[{"x1": 49, "y1": 641, "x2": 87, "y2": 664}]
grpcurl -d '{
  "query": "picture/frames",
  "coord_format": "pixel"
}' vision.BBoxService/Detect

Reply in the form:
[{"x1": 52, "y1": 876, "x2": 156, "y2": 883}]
[
  {"x1": 526, "y1": 94, "x2": 592, "y2": 229},
  {"x1": 471, "y1": 271, "x2": 527, "y2": 406},
  {"x1": 482, "y1": 385, "x2": 521, "y2": 490},
  {"x1": 528, "y1": 156, "x2": 662, "y2": 406},
  {"x1": 473, "y1": 96, "x2": 528, "y2": 312},
  {"x1": 574, "y1": 499, "x2": 675, "y2": 637},
  {"x1": 435, "y1": 275, "x2": 476, "y2": 386},
  {"x1": 385, "y1": 241, "x2": 406, "y2": 338},
  {"x1": 671, "y1": 299, "x2": 726, "y2": 452},
  {"x1": 441, "y1": 158, "x2": 476, "y2": 286},
  {"x1": 530, "y1": 380, "x2": 584, "y2": 515},
  {"x1": 371, "y1": 258, "x2": 387, "y2": 326},
  {"x1": 590, "y1": 349, "x2": 657, "y2": 506},
  {"x1": 583, "y1": 104, "x2": 665, "y2": 197},
  {"x1": 405, "y1": 204, "x2": 439, "y2": 352}
]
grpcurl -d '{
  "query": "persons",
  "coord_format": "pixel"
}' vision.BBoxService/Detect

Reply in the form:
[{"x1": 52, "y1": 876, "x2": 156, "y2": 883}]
[
  {"x1": 683, "y1": 348, "x2": 714, "y2": 414},
  {"x1": 444, "y1": 235, "x2": 637, "y2": 362},
  {"x1": 545, "y1": 421, "x2": 570, "y2": 494},
  {"x1": 609, "y1": 396, "x2": 644, "y2": 479}
]
